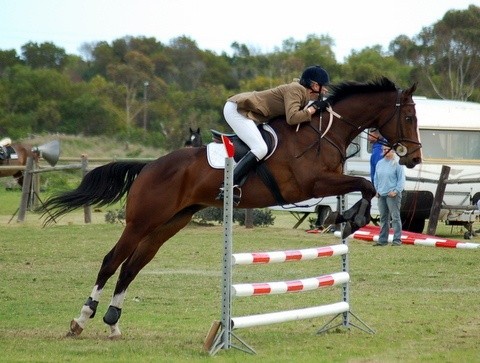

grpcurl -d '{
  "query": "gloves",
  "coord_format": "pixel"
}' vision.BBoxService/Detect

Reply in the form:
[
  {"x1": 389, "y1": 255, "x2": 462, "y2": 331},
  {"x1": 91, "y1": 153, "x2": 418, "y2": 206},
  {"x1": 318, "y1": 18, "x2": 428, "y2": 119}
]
[{"x1": 309, "y1": 100, "x2": 329, "y2": 115}]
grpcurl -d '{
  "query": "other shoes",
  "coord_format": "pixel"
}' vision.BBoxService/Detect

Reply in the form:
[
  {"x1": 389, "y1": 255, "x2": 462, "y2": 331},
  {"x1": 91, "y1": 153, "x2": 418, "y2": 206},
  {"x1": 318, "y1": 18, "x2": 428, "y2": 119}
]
[
  {"x1": 374, "y1": 241, "x2": 388, "y2": 246},
  {"x1": 392, "y1": 242, "x2": 401, "y2": 246}
]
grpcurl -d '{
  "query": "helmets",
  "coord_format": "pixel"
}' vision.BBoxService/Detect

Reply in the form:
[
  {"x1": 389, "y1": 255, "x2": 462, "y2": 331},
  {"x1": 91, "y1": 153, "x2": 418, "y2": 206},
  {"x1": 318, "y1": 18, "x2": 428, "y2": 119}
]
[{"x1": 302, "y1": 65, "x2": 329, "y2": 86}]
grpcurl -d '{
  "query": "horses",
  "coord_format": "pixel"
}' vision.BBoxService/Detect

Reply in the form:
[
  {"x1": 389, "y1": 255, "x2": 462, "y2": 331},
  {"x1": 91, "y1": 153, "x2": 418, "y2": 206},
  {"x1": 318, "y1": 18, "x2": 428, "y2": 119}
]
[
  {"x1": 34, "y1": 74, "x2": 423, "y2": 338},
  {"x1": 0, "y1": 142, "x2": 33, "y2": 208}
]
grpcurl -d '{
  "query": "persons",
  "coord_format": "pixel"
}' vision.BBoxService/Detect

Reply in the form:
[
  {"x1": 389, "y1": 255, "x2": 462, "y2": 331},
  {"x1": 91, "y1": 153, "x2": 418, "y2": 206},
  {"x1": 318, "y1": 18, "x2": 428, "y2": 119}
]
[
  {"x1": 369, "y1": 127, "x2": 388, "y2": 185},
  {"x1": 219, "y1": 66, "x2": 328, "y2": 201},
  {"x1": 374, "y1": 144, "x2": 405, "y2": 245}
]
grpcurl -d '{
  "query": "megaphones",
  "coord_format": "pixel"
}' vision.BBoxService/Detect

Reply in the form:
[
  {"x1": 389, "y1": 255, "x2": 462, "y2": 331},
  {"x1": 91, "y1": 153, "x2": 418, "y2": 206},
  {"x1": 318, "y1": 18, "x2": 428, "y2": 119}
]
[{"x1": 32, "y1": 139, "x2": 60, "y2": 167}]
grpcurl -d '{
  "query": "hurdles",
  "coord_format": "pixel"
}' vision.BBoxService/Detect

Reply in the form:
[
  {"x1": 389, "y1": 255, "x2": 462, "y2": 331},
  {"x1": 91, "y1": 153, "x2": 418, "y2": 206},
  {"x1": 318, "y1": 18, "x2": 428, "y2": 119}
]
[{"x1": 205, "y1": 157, "x2": 375, "y2": 356}]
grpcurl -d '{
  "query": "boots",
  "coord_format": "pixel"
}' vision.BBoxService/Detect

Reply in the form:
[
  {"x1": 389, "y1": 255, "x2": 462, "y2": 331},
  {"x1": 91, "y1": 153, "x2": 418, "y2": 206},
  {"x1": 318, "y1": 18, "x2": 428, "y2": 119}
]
[{"x1": 218, "y1": 151, "x2": 258, "y2": 199}]
